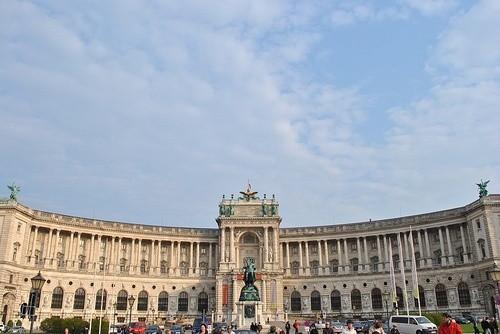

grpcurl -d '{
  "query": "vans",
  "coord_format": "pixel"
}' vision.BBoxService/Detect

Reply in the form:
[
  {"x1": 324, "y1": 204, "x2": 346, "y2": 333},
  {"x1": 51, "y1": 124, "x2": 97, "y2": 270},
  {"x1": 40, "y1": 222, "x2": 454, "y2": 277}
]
[
  {"x1": 390, "y1": 315, "x2": 438, "y2": 334},
  {"x1": 191, "y1": 317, "x2": 212, "y2": 334}
]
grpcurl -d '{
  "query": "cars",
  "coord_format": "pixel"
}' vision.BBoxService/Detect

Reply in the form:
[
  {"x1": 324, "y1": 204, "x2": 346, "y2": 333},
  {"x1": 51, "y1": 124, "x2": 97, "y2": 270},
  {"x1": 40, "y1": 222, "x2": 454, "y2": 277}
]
[
  {"x1": 170, "y1": 325, "x2": 183, "y2": 334},
  {"x1": 0, "y1": 324, "x2": 7, "y2": 333},
  {"x1": 315, "y1": 320, "x2": 368, "y2": 333},
  {"x1": 8, "y1": 326, "x2": 26, "y2": 334},
  {"x1": 127, "y1": 322, "x2": 147, "y2": 334},
  {"x1": 145, "y1": 325, "x2": 163, "y2": 334},
  {"x1": 454, "y1": 316, "x2": 471, "y2": 324}
]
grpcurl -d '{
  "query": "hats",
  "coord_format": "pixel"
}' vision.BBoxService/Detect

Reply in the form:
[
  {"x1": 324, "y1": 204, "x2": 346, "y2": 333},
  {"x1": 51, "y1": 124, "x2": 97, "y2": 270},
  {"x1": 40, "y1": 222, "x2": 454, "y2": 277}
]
[
  {"x1": 393, "y1": 325, "x2": 397, "y2": 327},
  {"x1": 346, "y1": 320, "x2": 353, "y2": 324}
]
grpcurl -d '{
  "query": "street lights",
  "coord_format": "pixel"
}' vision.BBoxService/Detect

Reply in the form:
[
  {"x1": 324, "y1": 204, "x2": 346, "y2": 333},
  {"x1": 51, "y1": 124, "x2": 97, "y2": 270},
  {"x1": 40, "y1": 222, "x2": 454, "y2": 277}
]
[
  {"x1": 29, "y1": 271, "x2": 46, "y2": 334},
  {"x1": 382, "y1": 290, "x2": 390, "y2": 333},
  {"x1": 128, "y1": 295, "x2": 135, "y2": 322},
  {"x1": 113, "y1": 302, "x2": 117, "y2": 334}
]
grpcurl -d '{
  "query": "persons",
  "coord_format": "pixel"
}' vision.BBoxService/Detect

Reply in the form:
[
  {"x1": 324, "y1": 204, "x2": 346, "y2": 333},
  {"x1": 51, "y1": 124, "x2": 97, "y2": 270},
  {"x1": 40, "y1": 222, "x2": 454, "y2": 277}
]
[
  {"x1": 391, "y1": 325, "x2": 400, "y2": 334},
  {"x1": 199, "y1": 324, "x2": 208, "y2": 334},
  {"x1": 474, "y1": 320, "x2": 480, "y2": 334},
  {"x1": 369, "y1": 320, "x2": 385, "y2": 334},
  {"x1": 481, "y1": 316, "x2": 499, "y2": 334},
  {"x1": 223, "y1": 325, "x2": 235, "y2": 334},
  {"x1": 285, "y1": 321, "x2": 291, "y2": 334},
  {"x1": 269, "y1": 326, "x2": 278, "y2": 334},
  {"x1": 342, "y1": 320, "x2": 357, "y2": 334},
  {"x1": 323, "y1": 323, "x2": 333, "y2": 334},
  {"x1": 437, "y1": 312, "x2": 463, "y2": 334},
  {"x1": 251, "y1": 321, "x2": 263, "y2": 333},
  {"x1": 294, "y1": 320, "x2": 299, "y2": 332},
  {"x1": 64, "y1": 328, "x2": 70, "y2": 334},
  {"x1": 302, "y1": 320, "x2": 311, "y2": 331}
]
